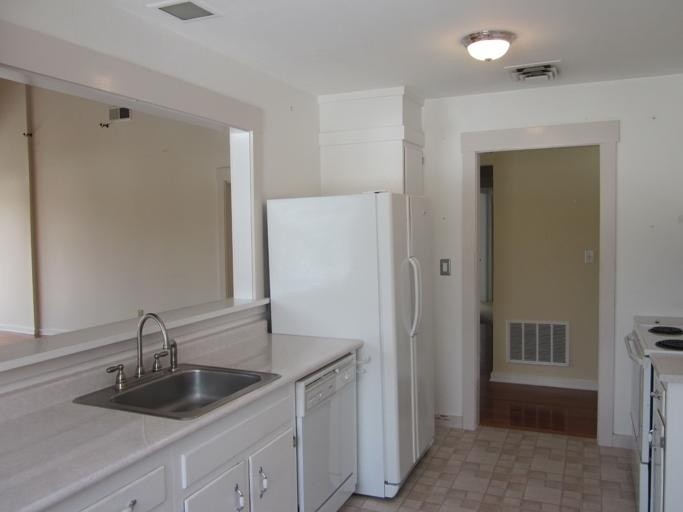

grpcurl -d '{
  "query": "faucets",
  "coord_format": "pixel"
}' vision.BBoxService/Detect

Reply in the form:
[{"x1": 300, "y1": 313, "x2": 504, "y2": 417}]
[{"x1": 135, "y1": 312, "x2": 171, "y2": 379}]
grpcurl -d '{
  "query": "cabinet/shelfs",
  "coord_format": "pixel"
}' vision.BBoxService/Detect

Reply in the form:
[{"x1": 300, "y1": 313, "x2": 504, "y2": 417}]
[
  {"x1": 80, "y1": 431, "x2": 298, "y2": 512},
  {"x1": 650, "y1": 353, "x2": 683, "y2": 512}
]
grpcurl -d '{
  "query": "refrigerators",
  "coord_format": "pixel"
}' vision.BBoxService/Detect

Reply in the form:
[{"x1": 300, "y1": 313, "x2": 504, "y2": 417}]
[{"x1": 261, "y1": 191, "x2": 434, "y2": 501}]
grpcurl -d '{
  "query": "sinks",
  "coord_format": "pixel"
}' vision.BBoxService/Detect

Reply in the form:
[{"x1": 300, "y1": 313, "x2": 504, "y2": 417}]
[{"x1": 111, "y1": 368, "x2": 262, "y2": 414}]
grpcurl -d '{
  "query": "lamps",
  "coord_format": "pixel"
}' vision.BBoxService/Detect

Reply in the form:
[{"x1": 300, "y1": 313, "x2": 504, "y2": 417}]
[{"x1": 462, "y1": 30, "x2": 516, "y2": 62}]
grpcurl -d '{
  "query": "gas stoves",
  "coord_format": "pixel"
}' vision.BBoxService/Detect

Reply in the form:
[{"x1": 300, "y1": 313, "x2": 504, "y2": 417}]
[{"x1": 631, "y1": 313, "x2": 682, "y2": 355}]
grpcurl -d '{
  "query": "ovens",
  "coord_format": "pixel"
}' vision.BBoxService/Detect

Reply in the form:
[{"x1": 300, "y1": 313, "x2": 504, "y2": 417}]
[{"x1": 621, "y1": 330, "x2": 652, "y2": 512}]
